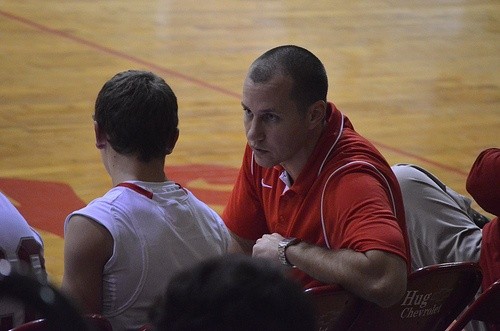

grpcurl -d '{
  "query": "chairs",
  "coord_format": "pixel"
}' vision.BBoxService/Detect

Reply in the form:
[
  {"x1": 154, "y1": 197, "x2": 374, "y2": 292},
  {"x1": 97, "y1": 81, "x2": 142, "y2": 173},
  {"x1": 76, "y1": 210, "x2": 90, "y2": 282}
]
[{"x1": 304, "y1": 260, "x2": 500, "y2": 331}]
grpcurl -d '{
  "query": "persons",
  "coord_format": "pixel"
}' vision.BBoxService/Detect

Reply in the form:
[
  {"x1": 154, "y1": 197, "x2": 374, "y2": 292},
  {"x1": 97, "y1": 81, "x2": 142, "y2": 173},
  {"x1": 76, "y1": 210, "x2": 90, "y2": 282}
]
[
  {"x1": 60, "y1": 68, "x2": 238, "y2": 330},
  {"x1": 219, "y1": 44, "x2": 412, "y2": 310},
  {"x1": 386, "y1": 145, "x2": 499, "y2": 330}
]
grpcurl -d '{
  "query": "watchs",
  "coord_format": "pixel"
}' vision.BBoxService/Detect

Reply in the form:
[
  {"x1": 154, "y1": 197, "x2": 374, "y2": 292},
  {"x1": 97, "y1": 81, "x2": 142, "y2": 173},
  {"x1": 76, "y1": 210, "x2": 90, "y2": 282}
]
[{"x1": 275, "y1": 236, "x2": 304, "y2": 268}]
